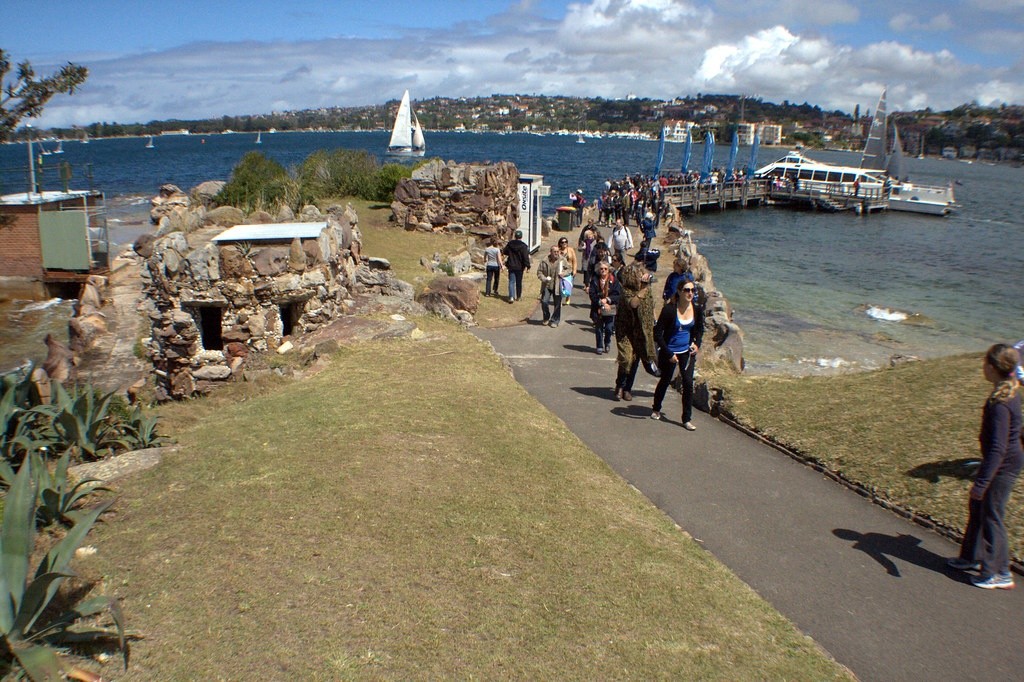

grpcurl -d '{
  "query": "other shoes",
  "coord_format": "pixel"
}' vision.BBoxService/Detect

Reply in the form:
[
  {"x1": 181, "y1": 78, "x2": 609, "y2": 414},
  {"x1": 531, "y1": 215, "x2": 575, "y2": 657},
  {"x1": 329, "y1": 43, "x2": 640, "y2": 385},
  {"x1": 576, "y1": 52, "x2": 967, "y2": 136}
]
[
  {"x1": 485, "y1": 292, "x2": 490, "y2": 297},
  {"x1": 508, "y1": 297, "x2": 514, "y2": 304},
  {"x1": 605, "y1": 343, "x2": 610, "y2": 352},
  {"x1": 493, "y1": 291, "x2": 499, "y2": 295},
  {"x1": 651, "y1": 412, "x2": 660, "y2": 421},
  {"x1": 542, "y1": 320, "x2": 549, "y2": 326},
  {"x1": 596, "y1": 347, "x2": 603, "y2": 355},
  {"x1": 684, "y1": 422, "x2": 696, "y2": 431},
  {"x1": 517, "y1": 298, "x2": 522, "y2": 301},
  {"x1": 565, "y1": 300, "x2": 570, "y2": 304},
  {"x1": 550, "y1": 323, "x2": 557, "y2": 328}
]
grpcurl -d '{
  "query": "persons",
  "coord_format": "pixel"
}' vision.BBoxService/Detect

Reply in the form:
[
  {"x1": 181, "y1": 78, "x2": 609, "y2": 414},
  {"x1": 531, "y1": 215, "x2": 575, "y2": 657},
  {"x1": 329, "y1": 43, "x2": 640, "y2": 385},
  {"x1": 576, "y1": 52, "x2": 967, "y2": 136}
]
[
  {"x1": 577, "y1": 230, "x2": 597, "y2": 293},
  {"x1": 644, "y1": 212, "x2": 657, "y2": 249},
  {"x1": 483, "y1": 234, "x2": 504, "y2": 297},
  {"x1": 650, "y1": 278, "x2": 704, "y2": 431},
  {"x1": 662, "y1": 259, "x2": 699, "y2": 306},
  {"x1": 502, "y1": 231, "x2": 531, "y2": 302},
  {"x1": 537, "y1": 165, "x2": 801, "y2": 355},
  {"x1": 941, "y1": 343, "x2": 1024, "y2": 590},
  {"x1": 614, "y1": 261, "x2": 656, "y2": 401},
  {"x1": 558, "y1": 237, "x2": 577, "y2": 307},
  {"x1": 588, "y1": 261, "x2": 618, "y2": 355},
  {"x1": 537, "y1": 245, "x2": 572, "y2": 328},
  {"x1": 584, "y1": 244, "x2": 613, "y2": 294},
  {"x1": 635, "y1": 241, "x2": 660, "y2": 272},
  {"x1": 572, "y1": 188, "x2": 587, "y2": 227},
  {"x1": 854, "y1": 178, "x2": 860, "y2": 196},
  {"x1": 578, "y1": 217, "x2": 598, "y2": 247},
  {"x1": 607, "y1": 219, "x2": 634, "y2": 254}
]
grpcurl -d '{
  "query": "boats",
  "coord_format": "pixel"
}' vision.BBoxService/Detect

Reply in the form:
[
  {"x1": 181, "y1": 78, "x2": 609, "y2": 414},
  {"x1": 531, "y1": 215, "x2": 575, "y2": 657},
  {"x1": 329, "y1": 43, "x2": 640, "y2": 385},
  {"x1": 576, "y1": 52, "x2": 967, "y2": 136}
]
[{"x1": 752, "y1": 150, "x2": 963, "y2": 216}]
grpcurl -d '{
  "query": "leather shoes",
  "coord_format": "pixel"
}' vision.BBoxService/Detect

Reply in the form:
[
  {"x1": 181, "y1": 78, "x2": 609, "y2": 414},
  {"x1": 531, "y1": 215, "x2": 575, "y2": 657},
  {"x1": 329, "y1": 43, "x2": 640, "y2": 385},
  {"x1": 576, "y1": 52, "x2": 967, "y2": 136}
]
[
  {"x1": 623, "y1": 391, "x2": 632, "y2": 401},
  {"x1": 615, "y1": 386, "x2": 622, "y2": 401}
]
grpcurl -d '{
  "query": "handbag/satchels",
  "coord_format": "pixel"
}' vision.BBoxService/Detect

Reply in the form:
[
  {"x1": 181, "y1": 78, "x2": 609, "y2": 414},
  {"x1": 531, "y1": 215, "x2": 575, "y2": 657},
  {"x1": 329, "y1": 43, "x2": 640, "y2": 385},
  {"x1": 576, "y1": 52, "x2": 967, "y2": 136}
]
[
  {"x1": 598, "y1": 303, "x2": 616, "y2": 318},
  {"x1": 559, "y1": 274, "x2": 572, "y2": 297}
]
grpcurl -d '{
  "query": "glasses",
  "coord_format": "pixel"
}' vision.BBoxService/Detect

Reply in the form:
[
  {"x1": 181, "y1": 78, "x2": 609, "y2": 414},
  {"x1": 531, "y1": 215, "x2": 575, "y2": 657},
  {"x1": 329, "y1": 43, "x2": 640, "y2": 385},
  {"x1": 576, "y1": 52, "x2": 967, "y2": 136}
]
[
  {"x1": 560, "y1": 241, "x2": 567, "y2": 244},
  {"x1": 612, "y1": 258, "x2": 618, "y2": 262},
  {"x1": 600, "y1": 267, "x2": 607, "y2": 271},
  {"x1": 680, "y1": 287, "x2": 696, "y2": 292}
]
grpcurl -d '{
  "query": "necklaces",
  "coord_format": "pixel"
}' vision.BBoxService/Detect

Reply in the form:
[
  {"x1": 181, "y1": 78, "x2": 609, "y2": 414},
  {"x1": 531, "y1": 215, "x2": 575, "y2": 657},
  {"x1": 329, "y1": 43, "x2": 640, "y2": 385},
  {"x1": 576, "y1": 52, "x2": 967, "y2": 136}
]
[{"x1": 679, "y1": 324, "x2": 682, "y2": 331}]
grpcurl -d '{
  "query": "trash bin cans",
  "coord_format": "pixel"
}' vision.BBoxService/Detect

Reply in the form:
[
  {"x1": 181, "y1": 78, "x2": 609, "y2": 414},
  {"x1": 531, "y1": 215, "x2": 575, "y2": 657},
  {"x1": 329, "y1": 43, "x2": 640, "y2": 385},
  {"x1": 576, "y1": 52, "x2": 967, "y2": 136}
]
[{"x1": 555, "y1": 206, "x2": 577, "y2": 232}]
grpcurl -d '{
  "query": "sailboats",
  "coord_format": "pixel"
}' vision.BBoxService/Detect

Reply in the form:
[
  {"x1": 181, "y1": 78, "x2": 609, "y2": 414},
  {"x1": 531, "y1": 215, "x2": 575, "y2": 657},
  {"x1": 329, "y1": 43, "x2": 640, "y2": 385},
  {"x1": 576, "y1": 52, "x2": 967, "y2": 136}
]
[
  {"x1": 384, "y1": 88, "x2": 426, "y2": 157},
  {"x1": 918, "y1": 135, "x2": 924, "y2": 159},
  {"x1": 576, "y1": 131, "x2": 586, "y2": 144},
  {"x1": 145, "y1": 137, "x2": 155, "y2": 148},
  {"x1": 37, "y1": 137, "x2": 64, "y2": 155},
  {"x1": 80, "y1": 131, "x2": 90, "y2": 144},
  {"x1": 255, "y1": 130, "x2": 262, "y2": 144}
]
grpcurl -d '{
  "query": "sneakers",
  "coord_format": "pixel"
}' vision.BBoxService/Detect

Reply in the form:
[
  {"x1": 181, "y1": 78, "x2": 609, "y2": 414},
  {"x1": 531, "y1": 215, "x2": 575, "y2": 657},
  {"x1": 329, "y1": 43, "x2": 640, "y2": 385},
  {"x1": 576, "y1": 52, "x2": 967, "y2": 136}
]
[
  {"x1": 948, "y1": 556, "x2": 982, "y2": 571},
  {"x1": 970, "y1": 572, "x2": 1015, "y2": 589}
]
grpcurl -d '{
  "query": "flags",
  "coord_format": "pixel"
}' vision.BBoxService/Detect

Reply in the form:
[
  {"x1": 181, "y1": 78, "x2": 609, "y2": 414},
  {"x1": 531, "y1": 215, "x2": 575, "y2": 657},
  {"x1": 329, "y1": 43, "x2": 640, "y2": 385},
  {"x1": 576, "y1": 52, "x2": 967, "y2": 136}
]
[{"x1": 569, "y1": 193, "x2": 577, "y2": 200}]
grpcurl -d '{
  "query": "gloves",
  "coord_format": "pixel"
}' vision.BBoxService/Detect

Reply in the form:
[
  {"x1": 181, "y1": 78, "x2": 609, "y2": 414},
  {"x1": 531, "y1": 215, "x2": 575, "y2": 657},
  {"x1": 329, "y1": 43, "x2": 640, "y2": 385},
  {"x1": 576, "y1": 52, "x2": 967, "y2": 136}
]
[{"x1": 969, "y1": 478, "x2": 991, "y2": 501}]
